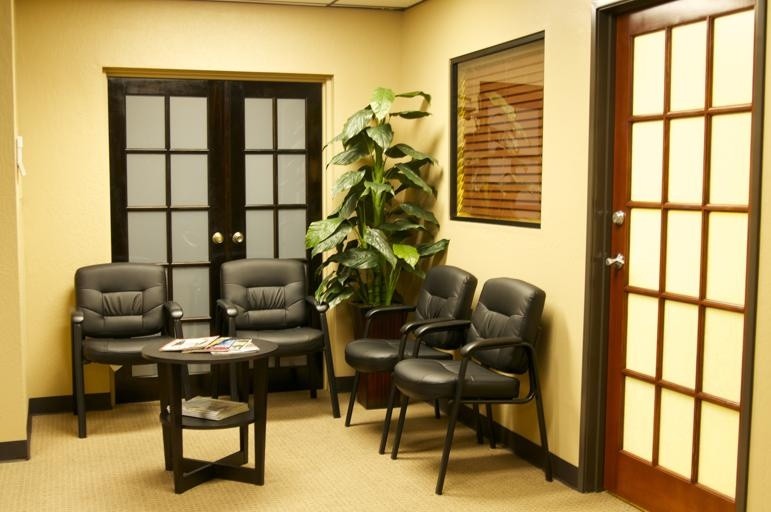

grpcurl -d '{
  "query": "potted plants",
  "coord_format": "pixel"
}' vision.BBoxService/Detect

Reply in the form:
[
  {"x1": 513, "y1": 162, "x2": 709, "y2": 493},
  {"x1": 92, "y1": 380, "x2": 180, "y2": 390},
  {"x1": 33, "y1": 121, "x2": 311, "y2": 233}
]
[{"x1": 305, "y1": 89, "x2": 449, "y2": 410}]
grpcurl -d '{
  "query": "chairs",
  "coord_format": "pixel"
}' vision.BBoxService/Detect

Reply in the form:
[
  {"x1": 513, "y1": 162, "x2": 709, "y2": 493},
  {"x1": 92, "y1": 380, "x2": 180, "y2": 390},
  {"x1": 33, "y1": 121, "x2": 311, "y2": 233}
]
[
  {"x1": 71, "y1": 262, "x2": 191, "y2": 438},
  {"x1": 390, "y1": 277, "x2": 553, "y2": 496},
  {"x1": 345, "y1": 265, "x2": 482, "y2": 454},
  {"x1": 210, "y1": 259, "x2": 341, "y2": 420}
]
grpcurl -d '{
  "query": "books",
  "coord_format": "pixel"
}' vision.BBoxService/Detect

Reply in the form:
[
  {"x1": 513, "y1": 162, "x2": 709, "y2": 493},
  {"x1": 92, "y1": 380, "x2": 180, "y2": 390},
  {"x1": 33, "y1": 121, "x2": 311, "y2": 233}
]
[
  {"x1": 166, "y1": 396, "x2": 248, "y2": 421},
  {"x1": 158, "y1": 335, "x2": 261, "y2": 356}
]
